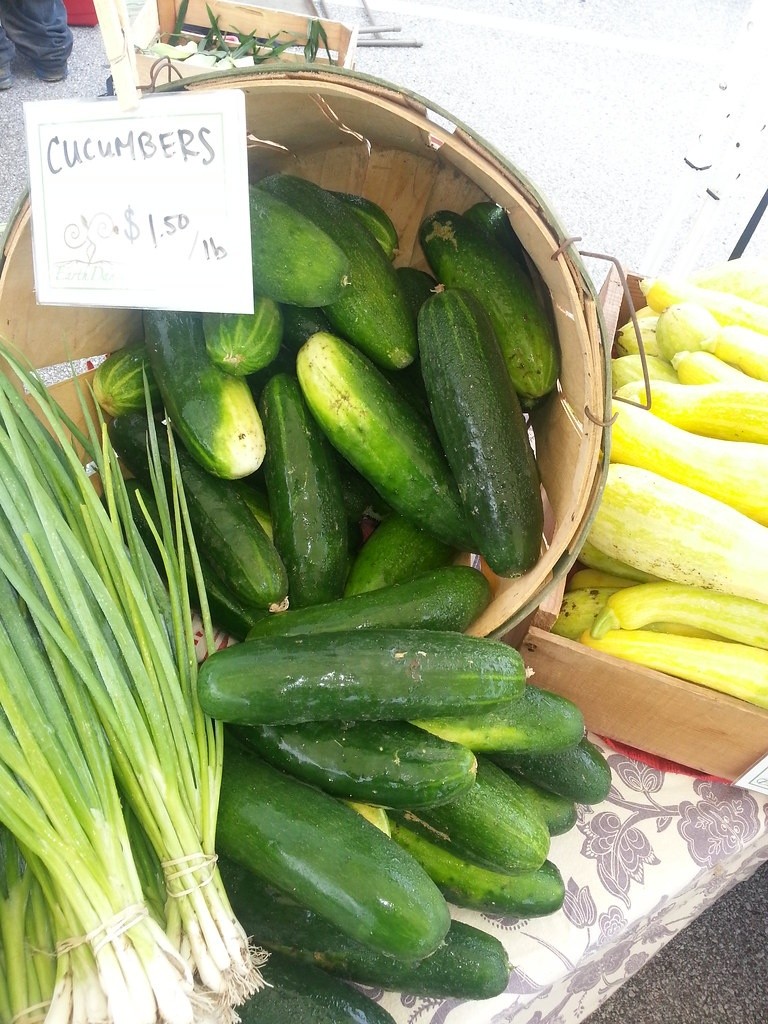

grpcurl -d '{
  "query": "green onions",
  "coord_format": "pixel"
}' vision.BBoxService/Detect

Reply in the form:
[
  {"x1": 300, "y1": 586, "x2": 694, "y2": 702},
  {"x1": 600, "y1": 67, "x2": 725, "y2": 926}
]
[{"x1": 0, "y1": 336, "x2": 273, "y2": 1024}]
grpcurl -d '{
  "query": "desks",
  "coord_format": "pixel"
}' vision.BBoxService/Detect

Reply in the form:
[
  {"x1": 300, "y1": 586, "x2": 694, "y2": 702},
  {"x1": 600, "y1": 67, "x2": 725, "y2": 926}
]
[{"x1": 349, "y1": 735, "x2": 768, "y2": 1024}]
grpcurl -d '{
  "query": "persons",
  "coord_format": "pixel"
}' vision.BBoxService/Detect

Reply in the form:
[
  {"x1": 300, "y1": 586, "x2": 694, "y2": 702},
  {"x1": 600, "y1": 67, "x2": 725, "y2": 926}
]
[{"x1": 0, "y1": 0, "x2": 73, "y2": 89}]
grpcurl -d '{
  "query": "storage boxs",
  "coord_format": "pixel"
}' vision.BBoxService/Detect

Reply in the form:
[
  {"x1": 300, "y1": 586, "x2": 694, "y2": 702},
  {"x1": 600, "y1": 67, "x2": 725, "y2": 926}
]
[
  {"x1": 130, "y1": 0, "x2": 359, "y2": 95},
  {"x1": 503, "y1": 573, "x2": 768, "y2": 795}
]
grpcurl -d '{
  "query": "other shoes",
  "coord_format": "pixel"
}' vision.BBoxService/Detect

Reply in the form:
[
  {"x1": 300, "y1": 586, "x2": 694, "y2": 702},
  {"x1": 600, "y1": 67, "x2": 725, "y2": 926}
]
[
  {"x1": 0, "y1": 63, "x2": 13, "y2": 90},
  {"x1": 16, "y1": 47, "x2": 69, "y2": 82}
]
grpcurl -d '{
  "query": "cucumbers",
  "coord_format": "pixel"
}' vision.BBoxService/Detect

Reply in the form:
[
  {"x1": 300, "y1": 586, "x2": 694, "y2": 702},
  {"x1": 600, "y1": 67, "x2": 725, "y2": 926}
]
[{"x1": 87, "y1": 176, "x2": 608, "y2": 1024}]
